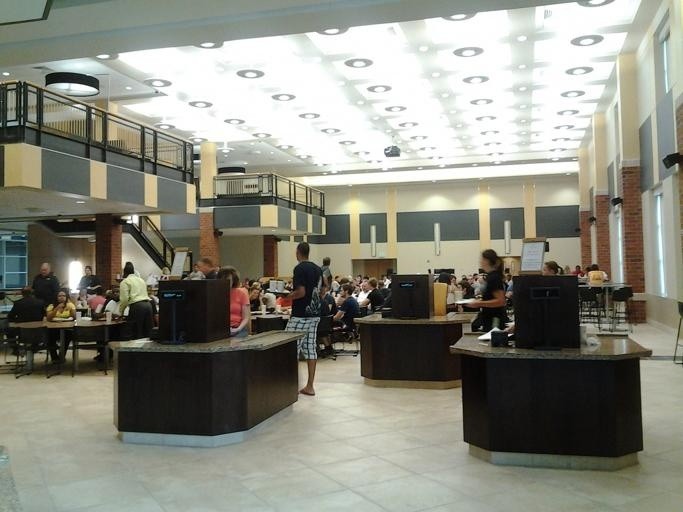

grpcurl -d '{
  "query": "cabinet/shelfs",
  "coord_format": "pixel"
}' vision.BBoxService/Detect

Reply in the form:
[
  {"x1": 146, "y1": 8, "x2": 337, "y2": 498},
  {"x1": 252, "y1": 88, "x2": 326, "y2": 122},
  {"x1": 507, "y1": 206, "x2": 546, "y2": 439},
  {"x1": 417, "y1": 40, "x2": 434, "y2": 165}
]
[{"x1": 0, "y1": 238, "x2": 28, "y2": 291}]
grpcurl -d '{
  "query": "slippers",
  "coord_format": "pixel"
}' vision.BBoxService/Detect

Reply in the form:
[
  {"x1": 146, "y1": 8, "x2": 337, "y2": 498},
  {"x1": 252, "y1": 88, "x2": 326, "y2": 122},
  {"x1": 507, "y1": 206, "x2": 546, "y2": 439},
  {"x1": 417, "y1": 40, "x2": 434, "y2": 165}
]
[{"x1": 300, "y1": 389, "x2": 315, "y2": 395}]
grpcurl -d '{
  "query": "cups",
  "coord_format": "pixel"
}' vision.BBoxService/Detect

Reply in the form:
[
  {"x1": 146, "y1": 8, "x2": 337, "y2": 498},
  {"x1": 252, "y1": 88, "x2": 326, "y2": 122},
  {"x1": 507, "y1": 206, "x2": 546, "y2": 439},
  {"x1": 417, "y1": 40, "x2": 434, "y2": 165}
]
[
  {"x1": 261, "y1": 305, "x2": 282, "y2": 317},
  {"x1": 269, "y1": 278, "x2": 284, "y2": 293},
  {"x1": 75, "y1": 311, "x2": 112, "y2": 325}
]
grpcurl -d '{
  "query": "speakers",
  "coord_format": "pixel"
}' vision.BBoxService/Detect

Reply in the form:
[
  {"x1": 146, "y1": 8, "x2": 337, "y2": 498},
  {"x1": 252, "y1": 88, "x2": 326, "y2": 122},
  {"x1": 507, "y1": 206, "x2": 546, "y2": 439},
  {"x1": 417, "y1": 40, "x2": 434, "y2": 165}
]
[
  {"x1": 588, "y1": 217, "x2": 595, "y2": 224},
  {"x1": 216, "y1": 231, "x2": 223, "y2": 237},
  {"x1": 611, "y1": 198, "x2": 622, "y2": 205}
]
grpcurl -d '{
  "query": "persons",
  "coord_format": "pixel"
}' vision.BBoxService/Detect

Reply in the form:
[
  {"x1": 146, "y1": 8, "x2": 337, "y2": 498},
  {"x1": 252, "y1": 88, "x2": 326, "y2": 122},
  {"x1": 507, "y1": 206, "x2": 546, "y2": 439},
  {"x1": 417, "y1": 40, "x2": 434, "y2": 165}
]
[
  {"x1": 8, "y1": 262, "x2": 150, "y2": 361},
  {"x1": 543, "y1": 261, "x2": 606, "y2": 282},
  {"x1": 283, "y1": 241, "x2": 325, "y2": 396},
  {"x1": 284, "y1": 257, "x2": 397, "y2": 360},
  {"x1": 429, "y1": 249, "x2": 513, "y2": 331},
  {"x1": 163, "y1": 256, "x2": 277, "y2": 337}
]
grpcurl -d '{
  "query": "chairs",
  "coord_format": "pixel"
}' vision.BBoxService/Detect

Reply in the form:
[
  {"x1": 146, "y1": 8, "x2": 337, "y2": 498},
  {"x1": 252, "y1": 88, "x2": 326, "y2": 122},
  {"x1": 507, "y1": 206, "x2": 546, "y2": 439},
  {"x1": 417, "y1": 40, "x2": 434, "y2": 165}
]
[
  {"x1": 448, "y1": 276, "x2": 683, "y2": 364},
  {"x1": 243, "y1": 274, "x2": 392, "y2": 360},
  {"x1": 0, "y1": 299, "x2": 157, "y2": 380}
]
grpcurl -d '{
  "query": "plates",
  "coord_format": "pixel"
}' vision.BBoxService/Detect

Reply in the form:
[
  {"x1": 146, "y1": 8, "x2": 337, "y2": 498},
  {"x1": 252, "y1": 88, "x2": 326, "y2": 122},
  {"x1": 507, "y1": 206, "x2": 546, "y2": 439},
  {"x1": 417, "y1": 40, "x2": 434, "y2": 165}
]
[{"x1": 454, "y1": 299, "x2": 474, "y2": 306}]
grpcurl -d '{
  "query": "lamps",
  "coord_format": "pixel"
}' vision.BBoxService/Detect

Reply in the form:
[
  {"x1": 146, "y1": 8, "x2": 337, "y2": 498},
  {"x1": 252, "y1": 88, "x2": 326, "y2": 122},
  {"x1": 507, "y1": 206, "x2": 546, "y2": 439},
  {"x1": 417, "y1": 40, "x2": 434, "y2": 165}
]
[
  {"x1": 573, "y1": 196, "x2": 622, "y2": 233},
  {"x1": 217, "y1": 165, "x2": 244, "y2": 178},
  {"x1": 41, "y1": 72, "x2": 100, "y2": 97}
]
